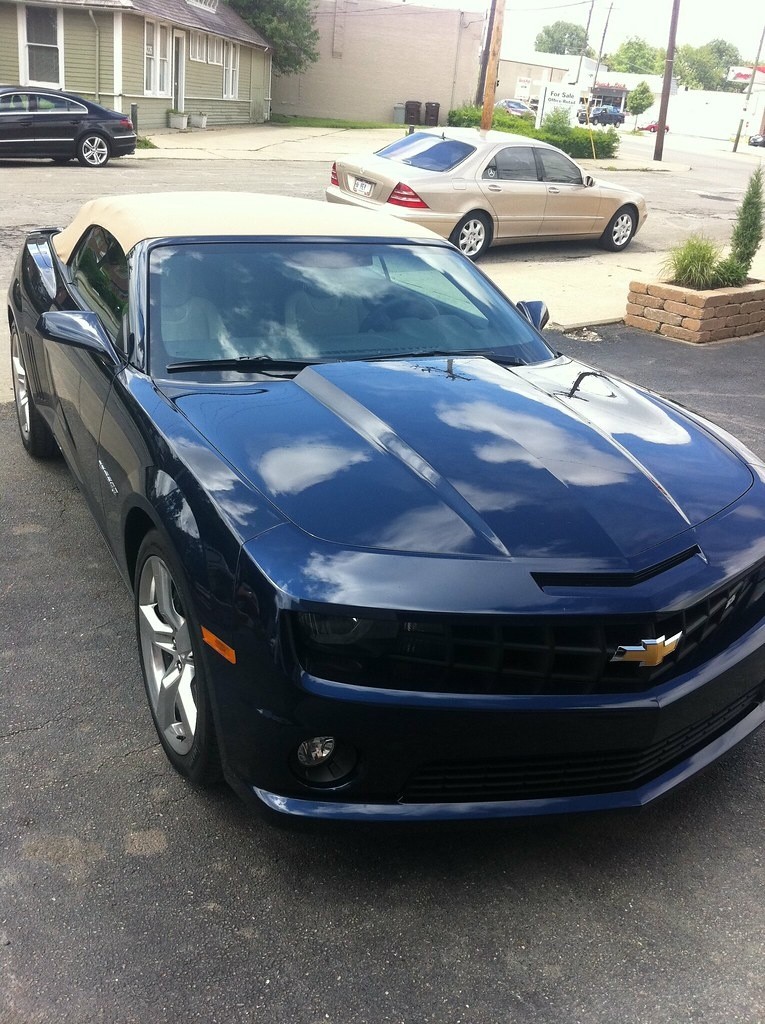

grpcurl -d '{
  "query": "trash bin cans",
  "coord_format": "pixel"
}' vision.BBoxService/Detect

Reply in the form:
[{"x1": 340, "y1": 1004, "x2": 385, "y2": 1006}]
[
  {"x1": 425, "y1": 102, "x2": 440, "y2": 126},
  {"x1": 405, "y1": 100, "x2": 422, "y2": 125},
  {"x1": 393, "y1": 102, "x2": 407, "y2": 125}
]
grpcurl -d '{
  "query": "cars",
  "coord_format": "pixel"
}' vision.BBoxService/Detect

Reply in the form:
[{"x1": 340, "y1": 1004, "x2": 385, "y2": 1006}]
[
  {"x1": 0, "y1": 84, "x2": 137, "y2": 167},
  {"x1": 495, "y1": 99, "x2": 537, "y2": 119},
  {"x1": 637, "y1": 119, "x2": 669, "y2": 133},
  {"x1": 8, "y1": 191, "x2": 765, "y2": 819},
  {"x1": 325, "y1": 126, "x2": 648, "y2": 262},
  {"x1": 578, "y1": 105, "x2": 625, "y2": 128}
]
